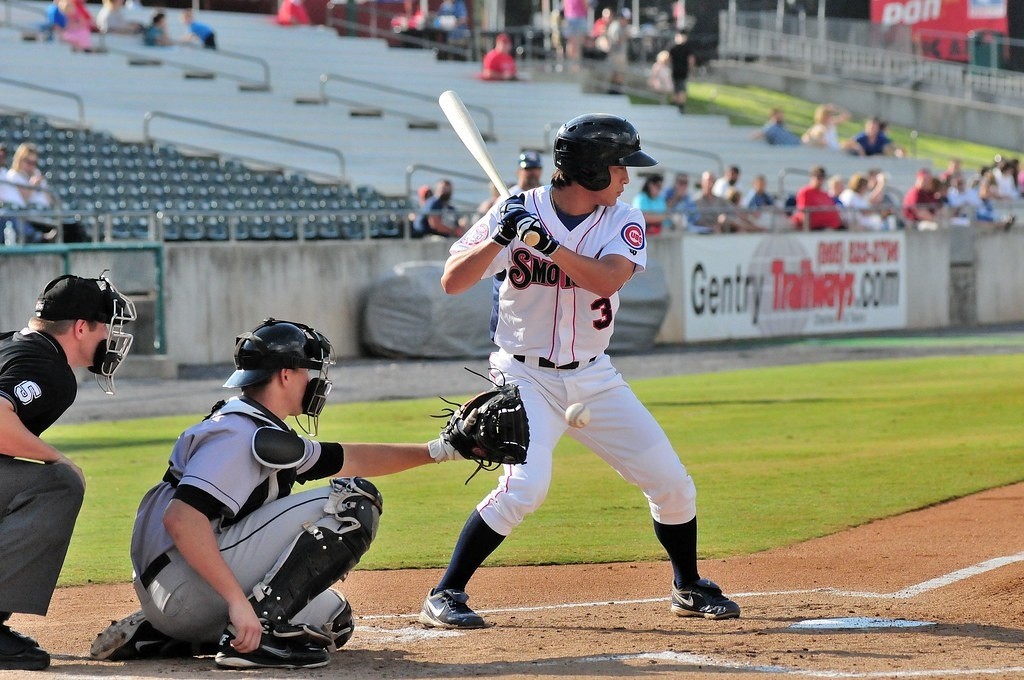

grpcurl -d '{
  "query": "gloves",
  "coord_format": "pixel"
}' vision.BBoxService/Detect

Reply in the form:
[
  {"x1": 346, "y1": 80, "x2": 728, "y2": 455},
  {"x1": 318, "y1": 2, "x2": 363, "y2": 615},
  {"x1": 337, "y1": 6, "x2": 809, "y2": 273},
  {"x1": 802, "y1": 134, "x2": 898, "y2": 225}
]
[
  {"x1": 514, "y1": 213, "x2": 560, "y2": 256},
  {"x1": 491, "y1": 193, "x2": 526, "y2": 246}
]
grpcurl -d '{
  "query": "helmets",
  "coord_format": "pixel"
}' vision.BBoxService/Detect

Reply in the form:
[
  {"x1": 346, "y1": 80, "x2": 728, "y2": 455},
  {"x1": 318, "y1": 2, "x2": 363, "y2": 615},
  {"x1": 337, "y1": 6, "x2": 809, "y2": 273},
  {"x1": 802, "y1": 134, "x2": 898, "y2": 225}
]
[
  {"x1": 222, "y1": 322, "x2": 307, "y2": 388},
  {"x1": 553, "y1": 113, "x2": 658, "y2": 191}
]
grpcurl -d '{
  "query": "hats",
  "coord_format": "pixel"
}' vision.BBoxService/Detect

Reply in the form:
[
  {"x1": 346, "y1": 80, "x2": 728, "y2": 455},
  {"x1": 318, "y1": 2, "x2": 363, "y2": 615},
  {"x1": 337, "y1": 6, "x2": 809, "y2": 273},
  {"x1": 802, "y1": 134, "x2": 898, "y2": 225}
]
[
  {"x1": 36, "y1": 275, "x2": 110, "y2": 323},
  {"x1": 519, "y1": 150, "x2": 540, "y2": 168}
]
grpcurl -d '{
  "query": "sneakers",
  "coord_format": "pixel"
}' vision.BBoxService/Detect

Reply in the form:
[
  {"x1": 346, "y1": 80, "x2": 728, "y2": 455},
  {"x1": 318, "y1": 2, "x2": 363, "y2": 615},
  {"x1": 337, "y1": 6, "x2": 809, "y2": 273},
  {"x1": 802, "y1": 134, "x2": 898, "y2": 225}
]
[
  {"x1": 671, "y1": 576, "x2": 740, "y2": 619},
  {"x1": 89, "y1": 610, "x2": 193, "y2": 661},
  {"x1": 419, "y1": 588, "x2": 484, "y2": 629},
  {"x1": 0, "y1": 623, "x2": 50, "y2": 670},
  {"x1": 215, "y1": 621, "x2": 331, "y2": 668}
]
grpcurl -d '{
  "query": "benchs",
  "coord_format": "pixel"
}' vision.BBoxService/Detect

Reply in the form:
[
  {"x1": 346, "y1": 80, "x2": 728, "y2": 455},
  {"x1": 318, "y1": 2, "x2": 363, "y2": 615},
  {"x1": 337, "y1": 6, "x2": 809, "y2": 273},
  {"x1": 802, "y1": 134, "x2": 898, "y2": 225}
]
[{"x1": 1, "y1": 3, "x2": 930, "y2": 240}]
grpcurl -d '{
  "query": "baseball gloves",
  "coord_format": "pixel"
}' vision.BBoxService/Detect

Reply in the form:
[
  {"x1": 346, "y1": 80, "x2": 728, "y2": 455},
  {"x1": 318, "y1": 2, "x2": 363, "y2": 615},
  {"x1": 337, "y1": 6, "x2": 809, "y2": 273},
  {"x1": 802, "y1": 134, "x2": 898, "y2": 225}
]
[{"x1": 429, "y1": 384, "x2": 531, "y2": 471}]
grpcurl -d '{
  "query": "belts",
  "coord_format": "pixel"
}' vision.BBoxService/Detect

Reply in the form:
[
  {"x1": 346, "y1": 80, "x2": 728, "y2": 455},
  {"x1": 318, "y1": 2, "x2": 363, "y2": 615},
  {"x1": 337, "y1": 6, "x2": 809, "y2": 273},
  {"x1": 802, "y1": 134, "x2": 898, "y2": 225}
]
[
  {"x1": 514, "y1": 355, "x2": 596, "y2": 369},
  {"x1": 140, "y1": 553, "x2": 170, "y2": 588}
]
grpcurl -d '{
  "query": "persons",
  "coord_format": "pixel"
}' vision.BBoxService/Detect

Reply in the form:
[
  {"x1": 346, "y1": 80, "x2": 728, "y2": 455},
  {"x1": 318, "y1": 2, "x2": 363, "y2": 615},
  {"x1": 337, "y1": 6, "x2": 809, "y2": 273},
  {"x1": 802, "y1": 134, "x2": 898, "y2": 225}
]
[
  {"x1": 551, "y1": 0, "x2": 697, "y2": 114},
  {"x1": 90, "y1": 317, "x2": 529, "y2": 671},
  {"x1": 482, "y1": 34, "x2": 517, "y2": 81},
  {"x1": 634, "y1": 153, "x2": 1023, "y2": 233},
  {"x1": 413, "y1": 177, "x2": 456, "y2": 237},
  {"x1": 276, "y1": 0, "x2": 311, "y2": 25},
  {"x1": 0, "y1": 141, "x2": 93, "y2": 244},
  {"x1": 391, "y1": 0, "x2": 470, "y2": 60},
  {"x1": 46, "y1": 0, "x2": 99, "y2": 54},
  {"x1": 97, "y1": 0, "x2": 216, "y2": 50},
  {"x1": 420, "y1": 112, "x2": 741, "y2": 627},
  {"x1": 473, "y1": 150, "x2": 545, "y2": 220},
  {"x1": 0, "y1": 274, "x2": 137, "y2": 670},
  {"x1": 753, "y1": 104, "x2": 906, "y2": 158}
]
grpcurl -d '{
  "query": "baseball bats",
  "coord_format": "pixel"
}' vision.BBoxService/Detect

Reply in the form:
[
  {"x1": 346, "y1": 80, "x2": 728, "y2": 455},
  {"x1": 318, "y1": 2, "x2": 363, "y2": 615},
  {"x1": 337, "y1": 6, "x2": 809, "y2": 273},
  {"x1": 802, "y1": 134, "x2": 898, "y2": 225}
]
[{"x1": 438, "y1": 90, "x2": 540, "y2": 246}]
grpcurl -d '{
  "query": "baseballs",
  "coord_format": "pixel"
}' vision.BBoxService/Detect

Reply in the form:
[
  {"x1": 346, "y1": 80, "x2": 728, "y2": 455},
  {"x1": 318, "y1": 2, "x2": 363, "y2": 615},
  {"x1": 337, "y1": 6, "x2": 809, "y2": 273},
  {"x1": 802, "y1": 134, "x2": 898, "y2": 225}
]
[{"x1": 565, "y1": 402, "x2": 591, "y2": 430}]
